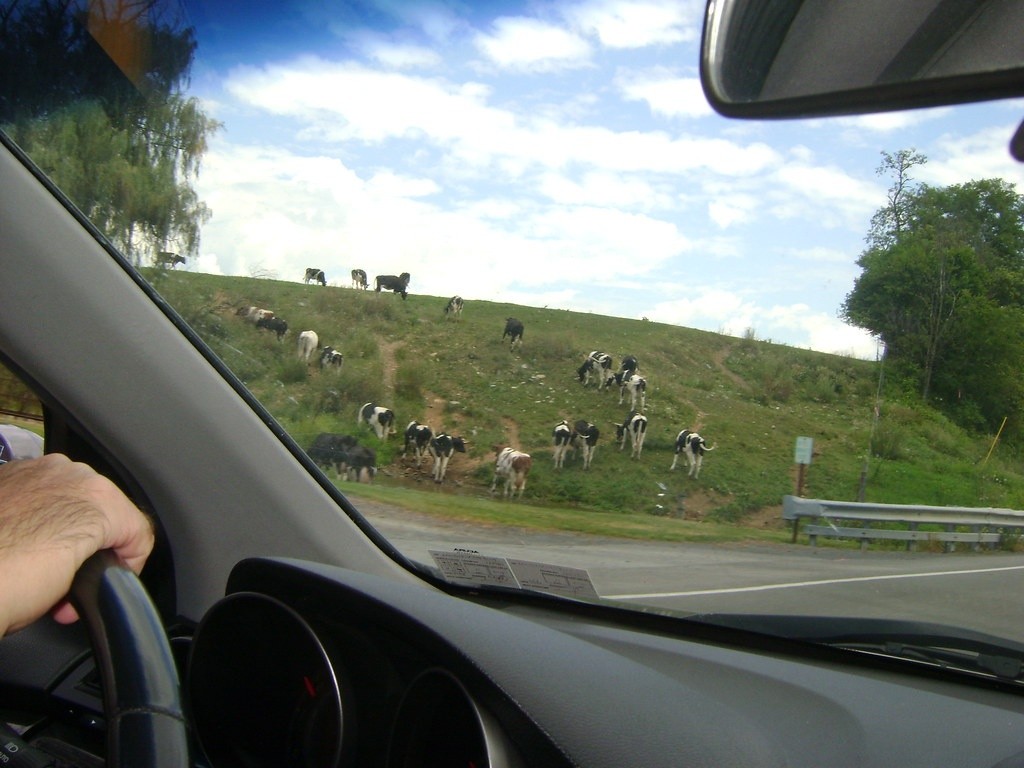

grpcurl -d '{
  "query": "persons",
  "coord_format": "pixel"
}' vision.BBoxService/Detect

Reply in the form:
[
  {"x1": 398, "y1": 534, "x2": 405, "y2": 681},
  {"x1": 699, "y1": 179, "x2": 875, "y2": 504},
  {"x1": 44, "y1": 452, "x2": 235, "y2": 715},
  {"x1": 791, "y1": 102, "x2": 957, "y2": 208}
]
[{"x1": 0, "y1": 452, "x2": 155, "y2": 644}]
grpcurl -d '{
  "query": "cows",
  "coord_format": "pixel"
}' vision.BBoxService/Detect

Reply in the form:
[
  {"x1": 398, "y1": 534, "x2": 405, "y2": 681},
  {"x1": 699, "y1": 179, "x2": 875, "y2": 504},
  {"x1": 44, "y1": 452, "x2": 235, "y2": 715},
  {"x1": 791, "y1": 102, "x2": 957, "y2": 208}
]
[
  {"x1": 401, "y1": 420, "x2": 432, "y2": 468},
  {"x1": 299, "y1": 329, "x2": 321, "y2": 365},
  {"x1": 358, "y1": 403, "x2": 394, "y2": 441},
  {"x1": 429, "y1": 433, "x2": 468, "y2": 482},
  {"x1": 572, "y1": 420, "x2": 601, "y2": 469},
  {"x1": 352, "y1": 269, "x2": 369, "y2": 290},
  {"x1": 551, "y1": 421, "x2": 572, "y2": 469},
  {"x1": 374, "y1": 272, "x2": 410, "y2": 300},
  {"x1": 490, "y1": 444, "x2": 530, "y2": 499},
  {"x1": 320, "y1": 345, "x2": 343, "y2": 369},
  {"x1": 307, "y1": 431, "x2": 377, "y2": 483},
  {"x1": 605, "y1": 355, "x2": 647, "y2": 412},
  {"x1": 237, "y1": 306, "x2": 288, "y2": 343},
  {"x1": 613, "y1": 411, "x2": 649, "y2": 459},
  {"x1": 577, "y1": 350, "x2": 613, "y2": 390},
  {"x1": 443, "y1": 295, "x2": 464, "y2": 317},
  {"x1": 303, "y1": 268, "x2": 328, "y2": 286},
  {"x1": 502, "y1": 317, "x2": 524, "y2": 353},
  {"x1": 669, "y1": 430, "x2": 715, "y2": 479}
]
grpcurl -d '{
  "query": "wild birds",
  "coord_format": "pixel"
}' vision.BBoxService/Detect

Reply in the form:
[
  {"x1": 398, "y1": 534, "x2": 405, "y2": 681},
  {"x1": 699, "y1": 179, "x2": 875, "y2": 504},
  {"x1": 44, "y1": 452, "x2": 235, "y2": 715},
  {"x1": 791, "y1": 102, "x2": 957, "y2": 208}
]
[{"x1": 155, "y1": 252, "x2": 185, "y2": 269}]
